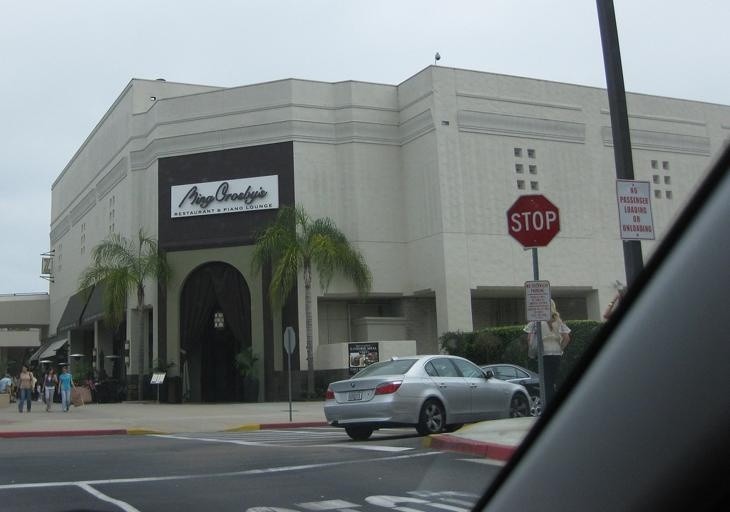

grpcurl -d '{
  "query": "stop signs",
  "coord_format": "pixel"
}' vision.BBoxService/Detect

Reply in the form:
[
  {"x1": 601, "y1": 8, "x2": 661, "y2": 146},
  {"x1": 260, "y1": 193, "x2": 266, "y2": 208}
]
[{"x1": 507, "y1": 193, "x2": 560, "y2": 249}]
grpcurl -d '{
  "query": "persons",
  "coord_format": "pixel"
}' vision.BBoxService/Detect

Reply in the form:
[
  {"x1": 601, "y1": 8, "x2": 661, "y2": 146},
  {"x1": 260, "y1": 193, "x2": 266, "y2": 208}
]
[
  {"x1": 364, "y1": 353, "x2": 375, "y2": 367},
  {"x1": 523, "y1": 299, "x2": 572, "y2": 406},
  {"x1": 603, "y1": 284, "x2": 627, "y2": 318},
  {"x1": 0, "y1": 365, "x2": 77, "y2": 412}
]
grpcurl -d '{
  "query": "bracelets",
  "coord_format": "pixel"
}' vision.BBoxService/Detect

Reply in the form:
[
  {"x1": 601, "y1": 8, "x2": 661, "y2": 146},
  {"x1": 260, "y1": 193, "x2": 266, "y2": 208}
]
[{"x1": 607, "y1": 298, "x2": 617, "y2": 306}]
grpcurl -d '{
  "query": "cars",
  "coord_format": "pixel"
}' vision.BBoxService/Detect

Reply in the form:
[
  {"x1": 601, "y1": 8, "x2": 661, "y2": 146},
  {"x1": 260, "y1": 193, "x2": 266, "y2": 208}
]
[
  {"x1": 469, "y1": 362, "x2": 542, "y2": 419},
  {"x1": 322, "y1": 354, "x2": 532, "y2": 442}
]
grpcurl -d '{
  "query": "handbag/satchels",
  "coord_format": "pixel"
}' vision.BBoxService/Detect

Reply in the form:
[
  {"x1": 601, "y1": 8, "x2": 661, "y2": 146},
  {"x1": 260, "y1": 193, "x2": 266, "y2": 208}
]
[
  {"x1": 71, "y1": 390, "x2": 84, "y2": 407},
  {"x1": 528, "y1": 321, "x2": 544, "y2": 359}
]
